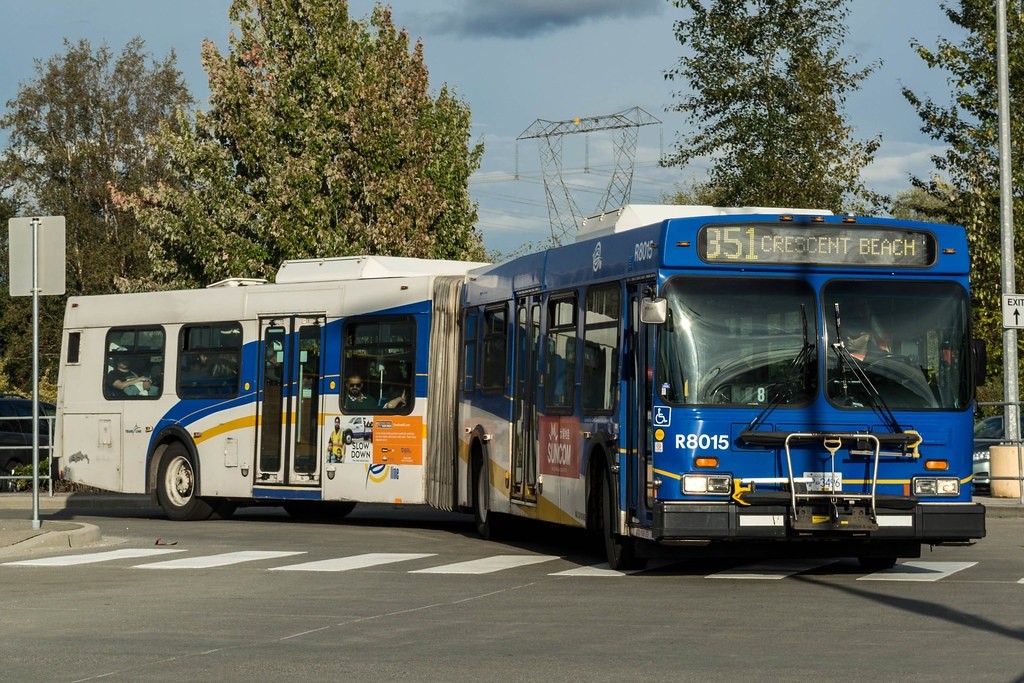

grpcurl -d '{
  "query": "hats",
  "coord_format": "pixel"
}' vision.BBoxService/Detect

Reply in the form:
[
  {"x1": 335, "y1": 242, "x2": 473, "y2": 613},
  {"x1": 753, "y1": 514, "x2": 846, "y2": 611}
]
[{"x1": 839, "y1": 316, "x2": 873, "y2": 335}]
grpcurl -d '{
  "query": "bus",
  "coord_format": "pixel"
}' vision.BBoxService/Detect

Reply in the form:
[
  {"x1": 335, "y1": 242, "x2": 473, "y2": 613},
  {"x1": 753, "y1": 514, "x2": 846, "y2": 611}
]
[{"x1": 52, "y1": 202, "x2": 990, "y2": 578}]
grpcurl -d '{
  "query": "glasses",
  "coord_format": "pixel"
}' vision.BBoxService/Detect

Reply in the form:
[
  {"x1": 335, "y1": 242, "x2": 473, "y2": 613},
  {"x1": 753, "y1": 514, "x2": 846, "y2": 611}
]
[
  {"x1": 847, "y1": 332, "x2": 866, "y2": 340},
  {"x1": 350, "y1": 383, "x2": 361, "y2": 387}
]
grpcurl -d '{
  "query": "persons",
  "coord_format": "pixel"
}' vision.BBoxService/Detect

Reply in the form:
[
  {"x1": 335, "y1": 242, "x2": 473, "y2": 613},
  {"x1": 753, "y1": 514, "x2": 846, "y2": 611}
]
[
  {"x1": 376, "y1": 353, "x2": 404, "y2": 385},
  {"x1": 103, "y1": 343, "x2": 291, "y2": 400},
  {"x1": 938, "y1": 337, "x2": 960, "y2": 388},
  {"x1": 342, "y1": 375, "x2": 407, "y2": 412},
  {"x1": 327, "y1": 416, "x2": 345, "y2": 463},
  {"x1": 840, "y1": 314, "x2": 887, "y2": 360},
  {"x1": 327, "y1": 440, "x2": 336, "y2": 464},
  {"x1": 336, "y1": 448, "x2": 344, "y2": 463}
]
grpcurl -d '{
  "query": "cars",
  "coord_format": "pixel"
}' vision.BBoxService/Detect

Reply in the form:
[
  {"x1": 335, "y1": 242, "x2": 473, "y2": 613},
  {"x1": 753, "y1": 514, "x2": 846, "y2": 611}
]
[
  {"x1": 1, "y1": 394, "x2": 57, "y2": 491},
  {"x1": 972, "y1": 414, "x2": 1024, "y2": 492}
]
